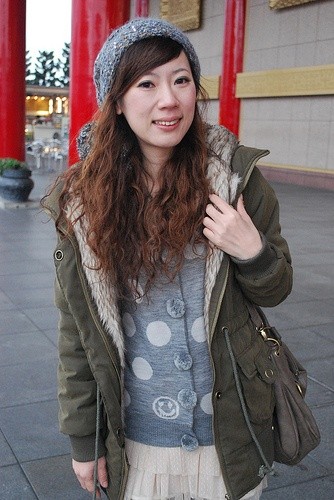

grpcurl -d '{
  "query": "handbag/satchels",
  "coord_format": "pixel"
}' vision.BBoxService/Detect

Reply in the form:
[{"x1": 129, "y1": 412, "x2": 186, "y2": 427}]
[{"x1": 258, "y1": 326, "x2": 321, "y2": 467}]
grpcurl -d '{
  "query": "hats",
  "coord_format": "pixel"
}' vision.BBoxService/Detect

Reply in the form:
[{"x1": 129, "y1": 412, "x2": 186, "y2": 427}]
[{"x1": 93, "y1": 18, "x2": 201, "y2": 110}]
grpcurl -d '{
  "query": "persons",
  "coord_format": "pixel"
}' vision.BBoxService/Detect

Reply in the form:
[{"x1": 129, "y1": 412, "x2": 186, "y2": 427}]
[{"x1": 39, "y1": 19, "x2": 292, "y2": 500}]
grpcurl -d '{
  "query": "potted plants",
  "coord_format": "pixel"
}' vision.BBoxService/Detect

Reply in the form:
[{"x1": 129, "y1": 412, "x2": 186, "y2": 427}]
[{"x1": 0, "y1": 157, "x2": 34, "y2": 202}]
